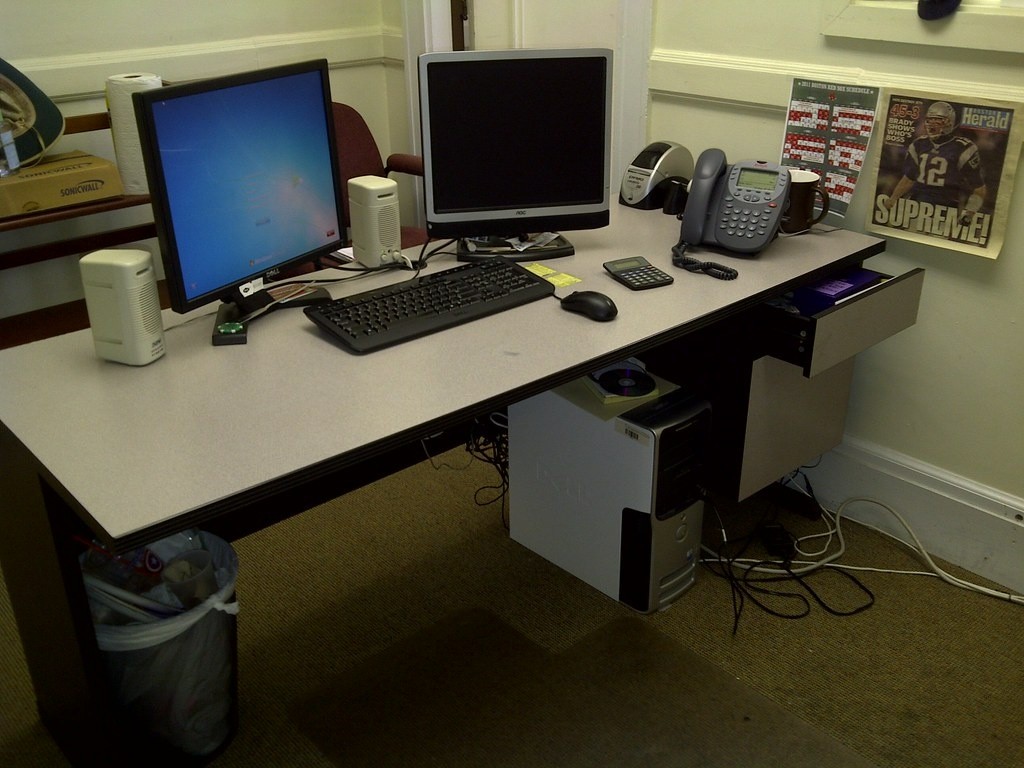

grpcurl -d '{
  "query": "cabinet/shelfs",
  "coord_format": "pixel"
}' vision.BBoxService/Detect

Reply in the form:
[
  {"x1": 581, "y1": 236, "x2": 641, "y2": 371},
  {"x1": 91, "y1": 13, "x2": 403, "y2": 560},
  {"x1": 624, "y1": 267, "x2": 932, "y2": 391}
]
[{"x1": 0, "y1": 193, "x2": 166, "y2": 321}]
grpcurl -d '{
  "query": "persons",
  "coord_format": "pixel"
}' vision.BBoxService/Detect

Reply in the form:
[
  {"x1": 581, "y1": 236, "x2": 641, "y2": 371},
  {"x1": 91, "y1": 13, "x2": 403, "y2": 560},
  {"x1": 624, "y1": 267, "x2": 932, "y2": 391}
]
[{"x1": 884, "y1": 102, "x2": 986, "y2": 226}]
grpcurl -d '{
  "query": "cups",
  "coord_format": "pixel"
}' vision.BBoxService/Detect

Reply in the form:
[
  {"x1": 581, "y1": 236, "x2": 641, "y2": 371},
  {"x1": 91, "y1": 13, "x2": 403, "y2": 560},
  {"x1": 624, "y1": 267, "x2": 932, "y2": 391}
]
[
  {"x1": 162, "y1": 549, "x2": 219, "y2": 610},
  {"x1": 777, "y1": 168, "x2": 830, "y2": 233}
]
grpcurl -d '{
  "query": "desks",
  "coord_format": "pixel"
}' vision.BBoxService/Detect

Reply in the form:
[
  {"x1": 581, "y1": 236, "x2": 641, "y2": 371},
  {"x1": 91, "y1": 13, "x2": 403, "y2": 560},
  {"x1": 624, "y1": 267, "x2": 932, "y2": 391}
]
[{"x1": 0, "y1": 192, "x2": 925, "y2": 768}]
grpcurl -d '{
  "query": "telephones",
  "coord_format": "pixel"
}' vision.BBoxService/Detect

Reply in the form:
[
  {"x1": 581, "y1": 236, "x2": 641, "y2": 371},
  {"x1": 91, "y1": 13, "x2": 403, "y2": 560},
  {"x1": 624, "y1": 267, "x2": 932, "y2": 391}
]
[{"x1": 676, "y1": 148, "x2": 793, "y2": 256}]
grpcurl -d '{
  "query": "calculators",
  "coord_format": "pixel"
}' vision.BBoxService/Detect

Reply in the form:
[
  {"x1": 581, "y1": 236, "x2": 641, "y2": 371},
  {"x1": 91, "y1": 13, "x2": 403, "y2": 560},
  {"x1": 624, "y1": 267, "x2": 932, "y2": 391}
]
[{"x1": 603, "y1": 256, "x2": 674, "y2": 291}]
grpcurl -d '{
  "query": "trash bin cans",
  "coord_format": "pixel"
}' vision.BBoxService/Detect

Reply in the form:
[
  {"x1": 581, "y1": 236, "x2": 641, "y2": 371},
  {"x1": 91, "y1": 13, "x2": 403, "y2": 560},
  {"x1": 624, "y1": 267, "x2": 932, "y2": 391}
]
[{"x1": 78, "y1": 531, "x2": 246, "y2": 768}]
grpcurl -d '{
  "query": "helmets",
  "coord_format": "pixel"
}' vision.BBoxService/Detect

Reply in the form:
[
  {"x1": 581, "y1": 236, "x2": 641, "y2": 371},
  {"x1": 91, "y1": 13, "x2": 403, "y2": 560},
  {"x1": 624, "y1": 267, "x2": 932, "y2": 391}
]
[{"x1": 925, "y1": 102, "x2": 955, "y2": 140}]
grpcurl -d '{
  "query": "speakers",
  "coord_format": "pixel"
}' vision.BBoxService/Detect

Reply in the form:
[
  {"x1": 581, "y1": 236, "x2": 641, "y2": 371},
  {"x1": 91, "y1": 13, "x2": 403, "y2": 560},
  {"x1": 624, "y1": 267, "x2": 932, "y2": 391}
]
[
  {"x1": 79, "y1": 249, "x2": 166, "y2": 366},
  {"x1": 347, "y1": 175, "x2": 400, "y2": 270}
]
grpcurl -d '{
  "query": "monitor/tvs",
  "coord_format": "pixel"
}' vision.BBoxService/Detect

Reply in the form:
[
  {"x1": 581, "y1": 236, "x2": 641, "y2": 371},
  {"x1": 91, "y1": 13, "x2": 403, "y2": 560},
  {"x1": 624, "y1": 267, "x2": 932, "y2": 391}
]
[
  {"x1": 132, "y1": 58, "x2": 345, "y2": 346},
  {"x1": 417, "y1": 49, "x2": 612, "y2": 261}
]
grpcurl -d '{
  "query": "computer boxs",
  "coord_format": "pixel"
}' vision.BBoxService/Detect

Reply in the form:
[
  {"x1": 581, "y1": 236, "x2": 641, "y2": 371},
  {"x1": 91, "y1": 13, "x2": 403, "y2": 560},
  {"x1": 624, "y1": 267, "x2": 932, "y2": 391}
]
[{"x1": 508, "y1": 382, "x2": 714, "y2": 613}]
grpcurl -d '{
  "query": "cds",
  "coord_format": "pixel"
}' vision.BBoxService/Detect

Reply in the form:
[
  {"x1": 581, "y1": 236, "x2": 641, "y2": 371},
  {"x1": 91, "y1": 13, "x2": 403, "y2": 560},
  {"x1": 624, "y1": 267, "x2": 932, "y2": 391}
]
[{"x1": 599, "y1": 369, "x2": 657, "y2": 398}]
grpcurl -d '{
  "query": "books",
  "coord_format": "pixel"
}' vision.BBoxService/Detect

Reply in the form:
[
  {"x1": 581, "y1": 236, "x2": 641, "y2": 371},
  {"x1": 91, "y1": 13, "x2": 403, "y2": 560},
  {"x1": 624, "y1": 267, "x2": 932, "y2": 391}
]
[{"x1": 549, "y1": 360, "x2": 681, "y2": 422}]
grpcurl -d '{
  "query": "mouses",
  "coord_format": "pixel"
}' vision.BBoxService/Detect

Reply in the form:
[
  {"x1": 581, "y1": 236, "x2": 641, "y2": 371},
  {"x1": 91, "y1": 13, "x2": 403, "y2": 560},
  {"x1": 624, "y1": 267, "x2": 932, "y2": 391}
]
[{"x1": 560, "y1": 291, "x2": 619, "y2": 322}]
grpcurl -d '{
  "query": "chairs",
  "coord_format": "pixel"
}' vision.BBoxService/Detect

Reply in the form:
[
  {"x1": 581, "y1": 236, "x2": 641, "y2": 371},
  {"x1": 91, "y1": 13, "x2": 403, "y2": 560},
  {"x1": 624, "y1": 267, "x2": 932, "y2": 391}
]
[{"x1": 279, "y1": 101, "x2": 429, "y2": 282}]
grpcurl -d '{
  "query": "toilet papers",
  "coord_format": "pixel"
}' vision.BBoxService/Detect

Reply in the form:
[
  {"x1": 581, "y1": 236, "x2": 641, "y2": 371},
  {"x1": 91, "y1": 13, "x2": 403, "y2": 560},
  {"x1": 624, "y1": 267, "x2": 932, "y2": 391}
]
[{"x1": 104, "y1": 72, "x2": 162, "y2": 196}]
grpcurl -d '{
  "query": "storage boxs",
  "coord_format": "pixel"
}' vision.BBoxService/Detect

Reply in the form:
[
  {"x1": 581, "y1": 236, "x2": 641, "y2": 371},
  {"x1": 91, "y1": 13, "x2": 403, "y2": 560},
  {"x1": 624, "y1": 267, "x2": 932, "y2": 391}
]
[{"x1": 1, "y1": 149, "x2": 125, "y2": 223}]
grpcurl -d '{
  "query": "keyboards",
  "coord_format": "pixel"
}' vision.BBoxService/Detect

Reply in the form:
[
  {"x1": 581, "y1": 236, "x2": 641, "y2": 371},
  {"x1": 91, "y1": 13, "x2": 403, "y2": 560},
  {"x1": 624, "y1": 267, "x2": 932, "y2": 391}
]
[{"x1": 303, "y1": 257, "x2": 554, "y2": 354}]
